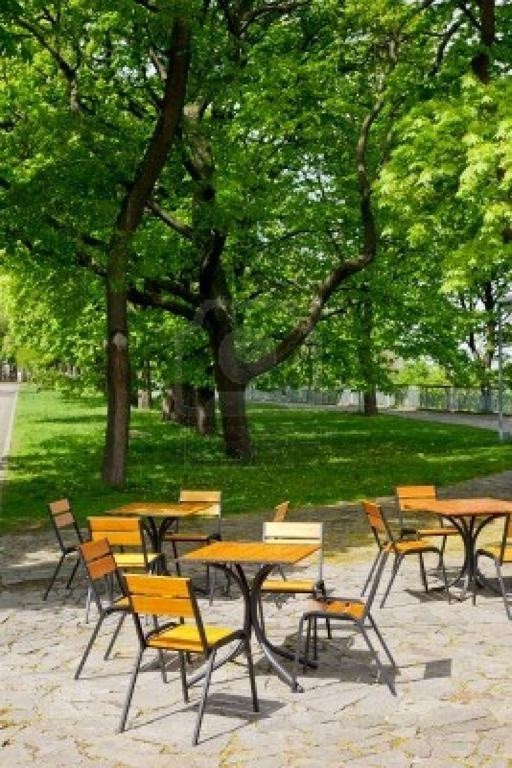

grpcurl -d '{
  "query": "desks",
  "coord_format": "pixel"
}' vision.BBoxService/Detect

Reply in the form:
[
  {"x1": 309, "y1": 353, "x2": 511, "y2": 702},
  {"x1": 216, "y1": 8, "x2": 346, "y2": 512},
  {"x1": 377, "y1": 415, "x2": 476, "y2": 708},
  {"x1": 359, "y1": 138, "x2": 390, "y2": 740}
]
[
  {"x1": 172, "y1": 541, "x2": 326, "y2": 693},
  {"x1": 404, "y1": 498, "x2": 512, "y2": 605},
  {"x1": 104, "y1": 502, "x2": 215, "y2": 575}
]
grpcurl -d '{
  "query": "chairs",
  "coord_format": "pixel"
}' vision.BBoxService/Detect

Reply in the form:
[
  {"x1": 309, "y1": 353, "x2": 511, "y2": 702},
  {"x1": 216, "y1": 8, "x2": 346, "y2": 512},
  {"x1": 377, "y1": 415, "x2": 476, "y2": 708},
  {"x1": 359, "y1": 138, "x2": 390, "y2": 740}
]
[
  {"x1": 73, "y1": 536, "x2": 191, "y2": 684},
  {"x1": 161, "y1": 490, "x2": 228, "y2": 608},
  {"x1": 473, "y1": 511, "x2": 512, "y2": 621},
  {"x1": 86, "y1": 515, "x2": 165, "y2": 578},
  {"x1": 250, "y1": 521, "x2": 331, "y2": 661},
  {"x1": 359, "y1": 500, "x2": 451, "y2": 609},
  {"x1": 42, "y1": 497, "x2": 82, "y2": 601},
  {"x1": 392, "y1": 485, "x2": 462, "y2": 572},
  {"x1": 117, "y1": 573, "x2": 260, "y2": 746},
  {"x1": 292, "y1": 541, "x2": 398, "y2": 697},
  {"x1": 224, "y1": 500, "x2": 295, "y2": 598}
]
